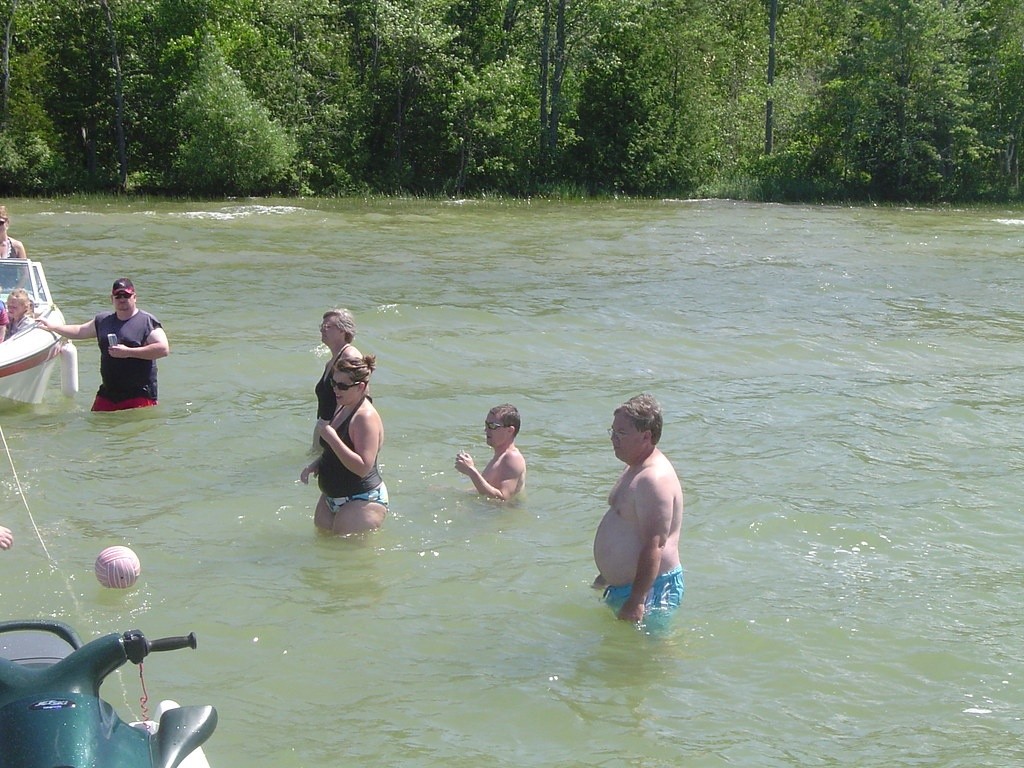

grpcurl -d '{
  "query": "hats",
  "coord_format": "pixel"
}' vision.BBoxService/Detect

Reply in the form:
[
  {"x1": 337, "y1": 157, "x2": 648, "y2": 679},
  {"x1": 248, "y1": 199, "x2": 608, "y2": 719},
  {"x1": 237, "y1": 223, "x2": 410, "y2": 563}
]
[{"x1": 112, "y1": 277, "x2": 133, "y2": 294}]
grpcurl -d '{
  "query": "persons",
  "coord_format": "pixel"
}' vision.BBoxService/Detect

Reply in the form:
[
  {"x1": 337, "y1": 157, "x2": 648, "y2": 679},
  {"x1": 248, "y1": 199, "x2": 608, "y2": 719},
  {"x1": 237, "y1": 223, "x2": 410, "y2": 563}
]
[
  {"x1": 5, "y1": 290, "x2": 34, "y2": 340},
  {"x1": 300, "y1": 356, "x2": 388, "y2": 531},
  {"x1": 0, "y1": 300, "x2": 9, "y2": 343},
  {"x1": 33, "y1": 279, "x2": 169, "y2": 411},
  {"x1": 0, "y1": 206, "x2": 29, "y2": 289},
  {"x1": 313, "y1": 309, "x2": 369, "y2": 448},
  {"x1": 593, "y1": 394, "x2": 684, "y2": 622},
  {"x1": 456, "y1": 403, "x2": 526, "y2": 501}
]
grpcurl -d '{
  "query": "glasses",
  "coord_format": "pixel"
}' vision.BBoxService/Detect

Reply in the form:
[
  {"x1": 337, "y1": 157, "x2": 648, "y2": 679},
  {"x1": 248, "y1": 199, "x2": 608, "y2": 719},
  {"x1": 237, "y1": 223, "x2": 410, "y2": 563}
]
[
  {"x1": 485, "y1": 421, "x2": 508, "y2": 431},
  {"x1": 0, "y1": 221, "x2": 6, "y2": 226},
  {"x1": 113, "y1": 293, "x2": 133, "y2": 299},
  {"x1": 319, "y1": 323, "x2": 334, "y2": 331},
  {"x1": 329, "y1": 378, "x2": 361, "y2": 390}
]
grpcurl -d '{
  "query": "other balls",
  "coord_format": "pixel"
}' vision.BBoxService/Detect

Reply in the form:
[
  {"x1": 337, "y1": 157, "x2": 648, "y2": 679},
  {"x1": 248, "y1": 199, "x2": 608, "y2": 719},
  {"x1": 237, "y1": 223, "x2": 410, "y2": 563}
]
[{"x1": 95, "y1": 545, "x2": 141, "y2": 588}]
